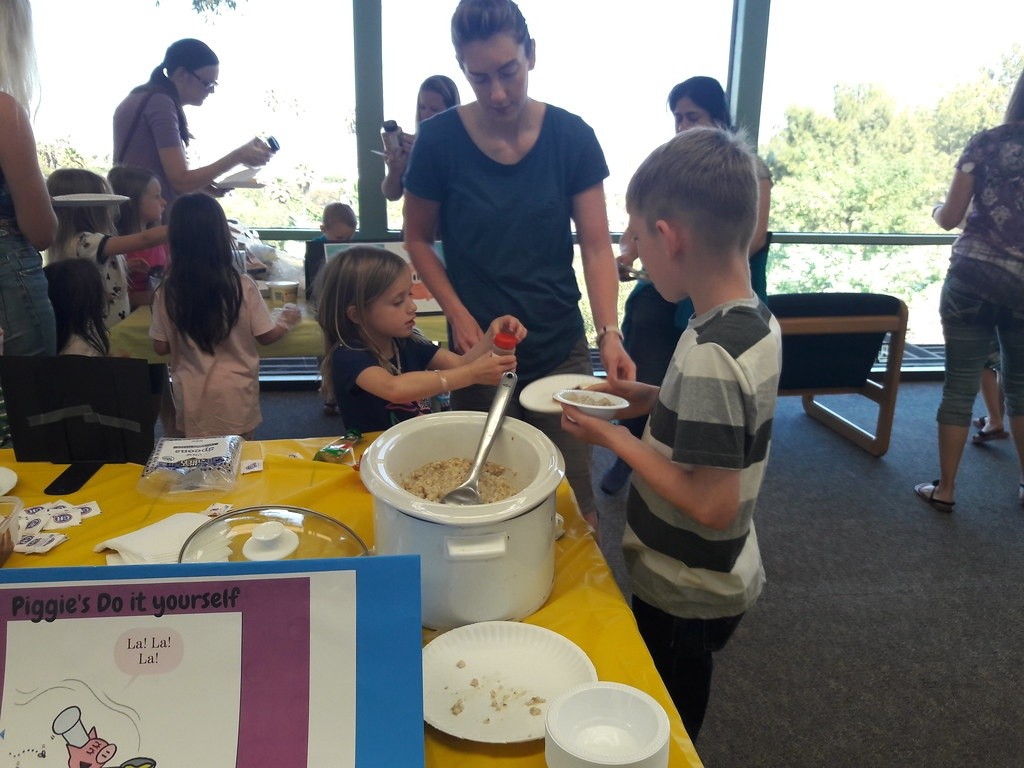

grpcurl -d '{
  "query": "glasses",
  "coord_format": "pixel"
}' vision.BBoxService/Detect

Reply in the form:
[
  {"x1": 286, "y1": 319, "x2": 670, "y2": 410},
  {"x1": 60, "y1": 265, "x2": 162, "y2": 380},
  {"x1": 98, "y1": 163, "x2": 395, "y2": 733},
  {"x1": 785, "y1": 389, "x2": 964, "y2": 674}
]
[{"x1": 183, "y1": 67, "x2": 220, "y2": 91}]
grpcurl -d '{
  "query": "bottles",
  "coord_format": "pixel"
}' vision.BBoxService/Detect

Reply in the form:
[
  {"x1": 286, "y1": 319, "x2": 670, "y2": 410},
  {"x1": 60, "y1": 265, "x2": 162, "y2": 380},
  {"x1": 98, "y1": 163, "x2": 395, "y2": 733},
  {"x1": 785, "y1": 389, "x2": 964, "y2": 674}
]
[
  {"x1": 380, "y1": 120, "x2": 404, "y2": 154},
  {"x1": 244, "y1": 136, "x2": 280, "y2": 170},
  {"x1": 492, "y1": 332, "x2": 516, "y2": 357}
]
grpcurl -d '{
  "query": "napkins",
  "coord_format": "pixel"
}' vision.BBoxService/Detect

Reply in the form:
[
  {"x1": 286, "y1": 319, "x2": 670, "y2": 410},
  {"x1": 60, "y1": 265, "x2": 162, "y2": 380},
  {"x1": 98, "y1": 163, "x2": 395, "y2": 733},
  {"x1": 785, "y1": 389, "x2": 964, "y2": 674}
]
[{"x1": 93, "y1": 513, "x2": 233, "y2": 565}]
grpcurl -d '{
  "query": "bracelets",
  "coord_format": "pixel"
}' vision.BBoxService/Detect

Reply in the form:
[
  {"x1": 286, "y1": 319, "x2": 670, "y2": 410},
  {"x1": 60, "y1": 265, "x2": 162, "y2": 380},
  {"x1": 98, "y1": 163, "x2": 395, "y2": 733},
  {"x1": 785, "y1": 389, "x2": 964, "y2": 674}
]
[{"x1": 596, "y1": 325, "x2": 624, "y2": 348}]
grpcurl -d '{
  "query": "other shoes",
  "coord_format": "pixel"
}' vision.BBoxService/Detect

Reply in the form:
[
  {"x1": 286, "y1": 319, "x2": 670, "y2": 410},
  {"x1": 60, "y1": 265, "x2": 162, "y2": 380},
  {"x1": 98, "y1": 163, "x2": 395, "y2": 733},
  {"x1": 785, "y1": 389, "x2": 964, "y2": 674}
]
[
  {"x1": 599, "y1": 459, "x2": 632, "y2": 493},
  {"x1": 323, "y1": 401, "x2": 340, "y2": 414}
]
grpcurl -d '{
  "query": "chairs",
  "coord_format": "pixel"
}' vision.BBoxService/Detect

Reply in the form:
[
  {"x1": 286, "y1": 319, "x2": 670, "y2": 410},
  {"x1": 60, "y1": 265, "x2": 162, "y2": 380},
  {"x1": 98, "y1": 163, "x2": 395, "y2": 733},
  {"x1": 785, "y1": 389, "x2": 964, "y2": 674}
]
[{"x1": 768, "y1": 293, "x2": 909, "y2": 458}]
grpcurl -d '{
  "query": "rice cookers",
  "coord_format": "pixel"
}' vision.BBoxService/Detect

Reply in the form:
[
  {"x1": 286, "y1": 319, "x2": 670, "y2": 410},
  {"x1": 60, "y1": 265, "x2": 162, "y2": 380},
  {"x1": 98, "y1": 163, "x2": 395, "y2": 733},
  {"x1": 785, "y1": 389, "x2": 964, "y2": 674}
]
[{"x1": 359, "y1": 410, "x2": 566, "y2": 631}]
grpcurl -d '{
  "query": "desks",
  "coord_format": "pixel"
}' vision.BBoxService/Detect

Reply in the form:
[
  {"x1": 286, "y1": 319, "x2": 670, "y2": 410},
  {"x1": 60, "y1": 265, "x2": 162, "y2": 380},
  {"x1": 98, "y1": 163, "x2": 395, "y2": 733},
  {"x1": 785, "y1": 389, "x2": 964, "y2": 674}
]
[
  {"x1": 0, "y1": 430, "x2": 705, "y2": 768},
  {"x1": 108, "y1": 303, "x2": 627, "y2": 356}
]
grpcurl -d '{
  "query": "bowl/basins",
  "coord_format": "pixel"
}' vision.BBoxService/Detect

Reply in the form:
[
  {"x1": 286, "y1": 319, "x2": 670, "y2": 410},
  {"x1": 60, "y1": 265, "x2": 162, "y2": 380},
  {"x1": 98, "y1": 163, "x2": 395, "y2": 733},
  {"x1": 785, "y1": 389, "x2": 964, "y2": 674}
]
[
  {"x1": 254, "y1": 281, "x2": 270, "y2": 298},
  {"x1": 545, "y1": 681, "x2": 671, "y2": 768},
  {"x1": 266, "y1": 281, "x2": 300, "y2": 309},
  {"x1": 552, "y1": 390, "x2": 630, "y2": 422},
  {"x1": 0, "y1": 496, "x2": 21, "y2": 568}
]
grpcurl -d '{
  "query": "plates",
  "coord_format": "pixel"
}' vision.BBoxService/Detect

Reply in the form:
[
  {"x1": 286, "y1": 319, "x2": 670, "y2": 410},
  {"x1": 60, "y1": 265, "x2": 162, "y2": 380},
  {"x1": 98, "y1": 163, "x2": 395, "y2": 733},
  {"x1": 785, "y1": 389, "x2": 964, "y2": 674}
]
[
  {"x1": 52, "y1": 194, "x2": 130, "y2": 206},
  {"x1": 519, "y1": 373, "x2": 607, "y2": 412},
  {"x1": 423, "y1": 620, "x2": 599, "y2": 744},
  {"x1": 0, "y1": 467, "x2": 18, "y2": 496}
]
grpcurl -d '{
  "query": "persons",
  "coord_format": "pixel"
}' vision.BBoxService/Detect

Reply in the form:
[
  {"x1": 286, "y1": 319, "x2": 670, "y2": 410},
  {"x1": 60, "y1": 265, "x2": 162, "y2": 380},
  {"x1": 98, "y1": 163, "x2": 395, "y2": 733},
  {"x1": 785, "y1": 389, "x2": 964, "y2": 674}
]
[
  {"x1": 114, "y1": 38, "x2": 272, "y2": 229},
  {"x1": 313, "y1": 245, "x2": 527, "y2": 433},
  {"x1": 0, "y1": 0, "x2": 59, "y2": 354},
  {"x1": 599, "y1": 76, "x2": 771, "y2": 495},
  {"x1": 403, "y1": 0, "x2": 637, "y2": 541},
  {"x1": 107, "y1": 164, "x2": 169, "y2": 313},
  {"x1": 43, "y1": 257, "x2": 110, "y2": 357},
  {"x1": 46, "y1": 169, "x2": 169, "y2": 328},
  {"x1": 150, "y1": 193, "x2": 301, "y2": 440},
  {"x1": 914, "y1": 67, "x2": 1024, "y2": 512},
  {"x1": 382, "y1": 75, "x2": 461, "y2": 200},
  {"x1": 560, "y1": 129, "x2": 782, "y2": 744},
  {"x1": 306, "y1": 203, "x2": 358, "y2": 301}
]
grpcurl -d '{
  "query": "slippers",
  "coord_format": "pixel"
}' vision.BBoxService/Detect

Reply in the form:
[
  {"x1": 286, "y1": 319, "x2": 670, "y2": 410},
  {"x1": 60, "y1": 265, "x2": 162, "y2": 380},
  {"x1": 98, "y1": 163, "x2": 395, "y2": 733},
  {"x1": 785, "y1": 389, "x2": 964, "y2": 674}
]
[
  {"x1": 972, "y1": 429, "x2": 1010, "y2": 442},
  {"x1": 973, "y1": 416, "x2": 988, "y2": 428}
]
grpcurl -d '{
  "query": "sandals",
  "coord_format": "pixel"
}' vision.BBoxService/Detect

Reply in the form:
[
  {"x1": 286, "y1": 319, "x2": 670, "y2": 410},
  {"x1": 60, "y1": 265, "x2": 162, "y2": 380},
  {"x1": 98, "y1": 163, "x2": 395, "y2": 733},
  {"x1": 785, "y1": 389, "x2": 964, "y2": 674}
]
[
  {"x1": 1019, "y1": 482, "x2": 1024, "y2": 505},
  {"x1": 915, "y1": 482, "x2": 955, "y2": 512}
]
